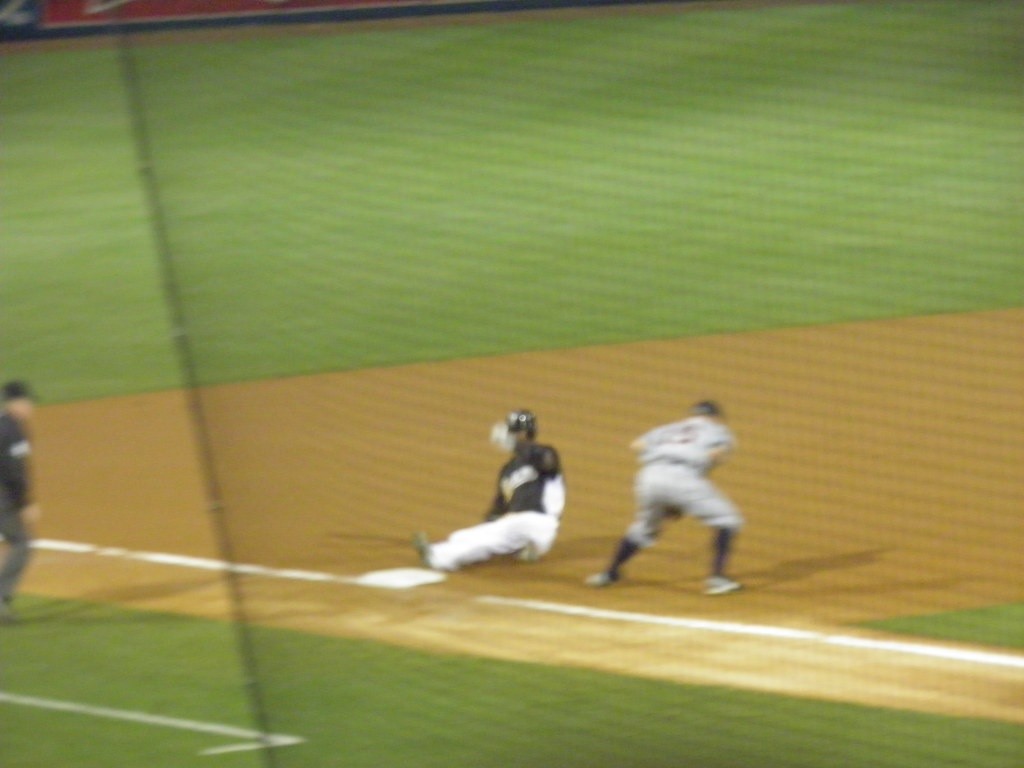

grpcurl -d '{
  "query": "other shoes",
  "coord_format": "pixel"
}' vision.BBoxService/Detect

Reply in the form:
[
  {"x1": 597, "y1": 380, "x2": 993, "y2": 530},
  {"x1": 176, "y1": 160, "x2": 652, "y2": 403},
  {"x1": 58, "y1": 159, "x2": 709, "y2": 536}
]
[
  {"x1": 703, "y1": 575, "x2": 745, "y2": 594},
  {"x1": 585, "y1": 572, "x2": 616, "y2": 587},
  {"x1": 413, "y1": 533, "x2": 431, "y2": 565}
]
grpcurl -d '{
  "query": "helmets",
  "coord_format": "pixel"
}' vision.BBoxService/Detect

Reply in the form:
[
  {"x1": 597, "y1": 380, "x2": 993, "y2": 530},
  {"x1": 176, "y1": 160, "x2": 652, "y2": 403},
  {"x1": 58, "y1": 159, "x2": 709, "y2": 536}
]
[
  {"x1": 3, "y1": 381, "x2": 33, "y2": 400},
  {"x1": 507, "y1": 410, "x2": 536, "y2": 439},
  {"x1": 690, "y1": 401, "x2": 718, "y2": 416}
]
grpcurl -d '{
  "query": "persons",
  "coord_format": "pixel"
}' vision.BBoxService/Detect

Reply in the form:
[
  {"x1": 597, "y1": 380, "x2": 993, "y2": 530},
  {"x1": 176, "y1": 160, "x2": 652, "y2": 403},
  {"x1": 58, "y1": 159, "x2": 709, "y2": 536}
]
[
  {"x1": 414, "y1": 409, "x2": 565, "y2": 571},
  {"x1": 0, "y1": 380, "x2": 40, "y2": 618},
  {"x1": 585, "y1": 401, "x2": 745, "y2": 595}
]
